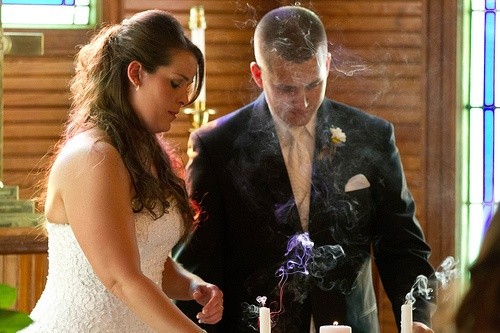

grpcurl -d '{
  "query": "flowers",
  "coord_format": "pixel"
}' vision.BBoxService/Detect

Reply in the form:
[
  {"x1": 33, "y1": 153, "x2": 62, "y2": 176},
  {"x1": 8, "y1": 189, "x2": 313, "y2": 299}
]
[{"x1": 328, "y1": 126, "x2": 345, "y2": 153}]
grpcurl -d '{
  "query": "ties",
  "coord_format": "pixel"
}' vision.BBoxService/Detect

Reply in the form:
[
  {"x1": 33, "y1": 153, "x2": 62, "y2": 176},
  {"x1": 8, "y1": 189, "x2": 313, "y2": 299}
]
[{"x1": 286, "y1": 125, "x2": 312, "y2": 234}]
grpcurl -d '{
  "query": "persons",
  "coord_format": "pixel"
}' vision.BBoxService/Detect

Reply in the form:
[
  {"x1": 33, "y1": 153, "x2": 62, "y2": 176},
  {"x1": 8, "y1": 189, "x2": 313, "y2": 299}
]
[
  {"x1": 27, "y1": 6, "x2": 225, "y2": 331},
  {"x1": 170, "y1": 4, "x2": 439, "y2": 332}
]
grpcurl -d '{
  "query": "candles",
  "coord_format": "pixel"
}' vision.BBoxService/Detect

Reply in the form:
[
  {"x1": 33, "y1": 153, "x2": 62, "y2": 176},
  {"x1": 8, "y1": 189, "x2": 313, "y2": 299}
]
[
  {"x1": 259, "y1": 306, "x2": 272, "y2": 333},
  {"x1": 400, "y1": 303, "x2": 413, "y2": 333},
  {"x1": 320, "y1": 324, "x2": 352, "y2": 333}
]
